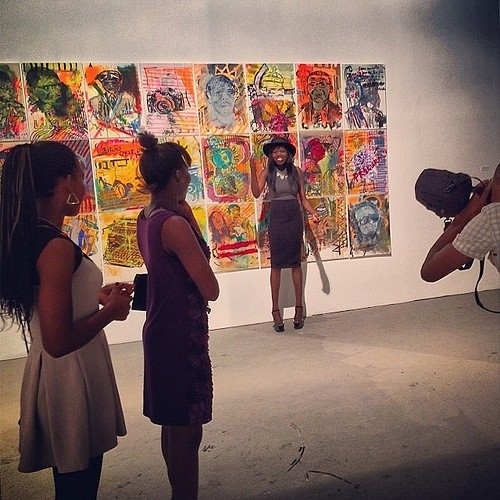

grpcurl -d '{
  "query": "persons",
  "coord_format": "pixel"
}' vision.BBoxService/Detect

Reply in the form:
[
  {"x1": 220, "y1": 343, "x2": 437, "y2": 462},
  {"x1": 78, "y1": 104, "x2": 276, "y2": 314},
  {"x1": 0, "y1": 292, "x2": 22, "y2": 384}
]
[
  {"x1": 249, "y1": 137, "x2": 322, "y2": 332},
  {"x1": 136, "y1": 131, "x2": 220, "y2": 500},
  {"x1": 0, "y1": 141, "x2": 134, "y2": 500},
  {"x1": 420, "y1": 161, "x2": 500, "y2": 283}
]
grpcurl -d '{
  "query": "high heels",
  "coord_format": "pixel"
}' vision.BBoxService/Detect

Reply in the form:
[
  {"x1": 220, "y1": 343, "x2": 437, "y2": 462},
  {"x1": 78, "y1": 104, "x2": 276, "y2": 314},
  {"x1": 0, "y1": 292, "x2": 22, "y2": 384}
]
[
  {"x1": 293, "y1": 305, "x2": 306, "y2": 329},
  {"x1": 271, "y1": 309, "x2": 285, "y2": 332}
]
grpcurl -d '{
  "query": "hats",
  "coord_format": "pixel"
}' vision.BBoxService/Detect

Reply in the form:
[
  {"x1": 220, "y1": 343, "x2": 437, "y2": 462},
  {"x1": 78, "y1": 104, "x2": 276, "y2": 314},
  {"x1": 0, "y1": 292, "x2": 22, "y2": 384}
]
[{"x1": 262, "y1": 137, "x2": 297, "y2": 157}]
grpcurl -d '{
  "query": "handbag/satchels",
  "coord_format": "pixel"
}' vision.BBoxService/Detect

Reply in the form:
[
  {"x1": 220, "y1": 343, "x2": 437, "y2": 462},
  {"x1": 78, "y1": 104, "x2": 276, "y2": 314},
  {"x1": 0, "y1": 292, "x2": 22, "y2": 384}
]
[{"x1": 131, "y1": 272, "x2": 149, "y2": 312}]
